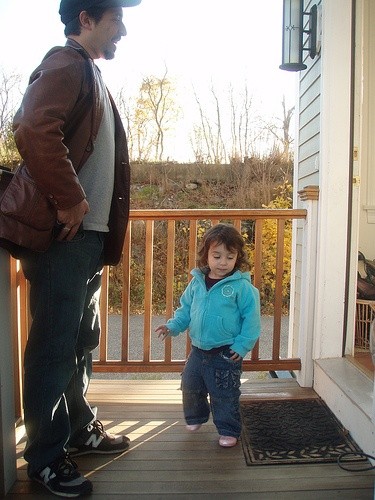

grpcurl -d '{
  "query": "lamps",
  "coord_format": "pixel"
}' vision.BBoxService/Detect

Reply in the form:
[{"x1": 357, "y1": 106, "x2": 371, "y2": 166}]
[{"x1": 278, "y1": 0, "x2": 317, "y2": 73}]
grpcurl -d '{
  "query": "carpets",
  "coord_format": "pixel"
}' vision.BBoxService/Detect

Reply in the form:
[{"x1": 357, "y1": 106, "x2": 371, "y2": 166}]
[{"x1": 240, "y1": 397, "x2": 366, "y2": 466}]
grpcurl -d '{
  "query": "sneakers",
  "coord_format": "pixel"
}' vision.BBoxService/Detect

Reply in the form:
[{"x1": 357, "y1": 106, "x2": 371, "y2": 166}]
[
  {"x1": 27, "y1": 448, "x2": 93, "y2": 497},
  {"x1": 66, "y1": 420, "x2": 131, "y2": 457}
]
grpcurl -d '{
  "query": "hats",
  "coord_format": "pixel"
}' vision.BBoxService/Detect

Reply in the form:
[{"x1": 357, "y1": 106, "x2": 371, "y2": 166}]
[{"x1": 59, "y1": 0, "x2": 141, "y2": 24}]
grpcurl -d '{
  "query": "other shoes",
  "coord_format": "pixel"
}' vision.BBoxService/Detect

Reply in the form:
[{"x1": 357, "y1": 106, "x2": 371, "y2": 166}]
[
  {"x1": 186, "y1": 423, "x2": 201, "y2": 431},
  {"x1": 219, "y1": 435, "x2": 237, "y2": 446}
]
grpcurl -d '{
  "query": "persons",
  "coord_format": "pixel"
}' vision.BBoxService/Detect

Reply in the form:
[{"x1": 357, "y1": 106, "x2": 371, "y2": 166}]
[
  {"x1": 153, "y1": 224, "x2": 261, "y2": 447},
  {"x1": 0, "y1": 1, "x2": 145, "y2": 500}
]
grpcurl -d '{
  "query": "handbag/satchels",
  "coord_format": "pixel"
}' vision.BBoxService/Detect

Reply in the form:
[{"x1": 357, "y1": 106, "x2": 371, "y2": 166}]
[{"x1": 0, "y1": 165, "x2": 28, "y2": 259}]
